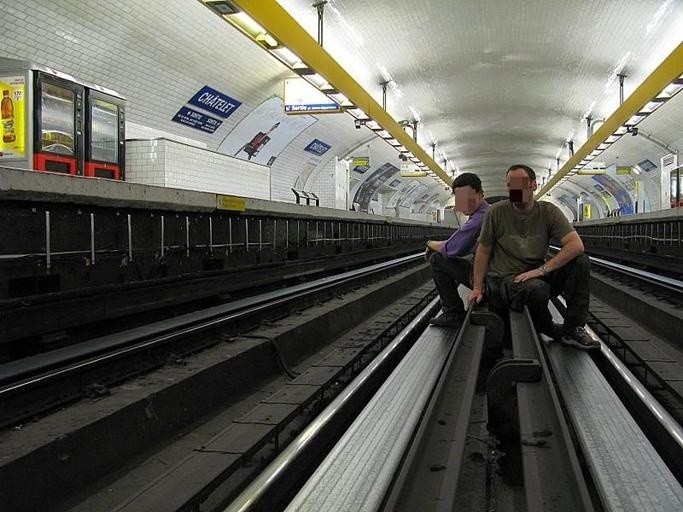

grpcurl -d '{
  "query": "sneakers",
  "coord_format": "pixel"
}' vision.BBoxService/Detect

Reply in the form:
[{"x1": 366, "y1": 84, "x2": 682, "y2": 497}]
[
  {"x1": 428, "y1": 311, "x2": 465, "y2": 327},
  {"x1": 560, "y1": 326, "x2": 603, "y2": 350},
  {"x1": 539, "y1": 321, "x2": 565, "y2": 341}
]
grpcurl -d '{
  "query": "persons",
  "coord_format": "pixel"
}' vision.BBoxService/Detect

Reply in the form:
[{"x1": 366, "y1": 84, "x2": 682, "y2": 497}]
[
  {"x1": 466, "y1": 165, "x2": 601, "y2": 351},
  {"x1": 424, "y1": 172, "x2": 494, "y2": 326}
]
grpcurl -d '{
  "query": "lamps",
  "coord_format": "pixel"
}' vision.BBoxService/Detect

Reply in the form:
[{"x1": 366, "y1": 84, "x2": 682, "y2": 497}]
[
  {"x1": 196, "y1": 0, "x2": 453, "y2": 192},
  {"x1": 542, "y1": 41, "x2": 683, "y2": 195}
]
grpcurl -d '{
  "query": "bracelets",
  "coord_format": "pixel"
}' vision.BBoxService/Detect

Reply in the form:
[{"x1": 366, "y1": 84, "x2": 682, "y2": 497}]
[{"x1": 539, "y1": 264, "x2": 550, "y2": 277}]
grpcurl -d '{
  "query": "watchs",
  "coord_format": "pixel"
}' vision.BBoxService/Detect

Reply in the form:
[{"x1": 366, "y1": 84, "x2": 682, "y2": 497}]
[{"x1": 426, "y1": 239, "x2": 431, "y2": 249}]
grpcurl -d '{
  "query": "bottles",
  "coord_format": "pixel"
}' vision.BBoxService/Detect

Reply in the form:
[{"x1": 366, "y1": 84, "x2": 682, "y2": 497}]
[{"x1": 1, "y1": 89, "x2": 16, "y2": 144}]
